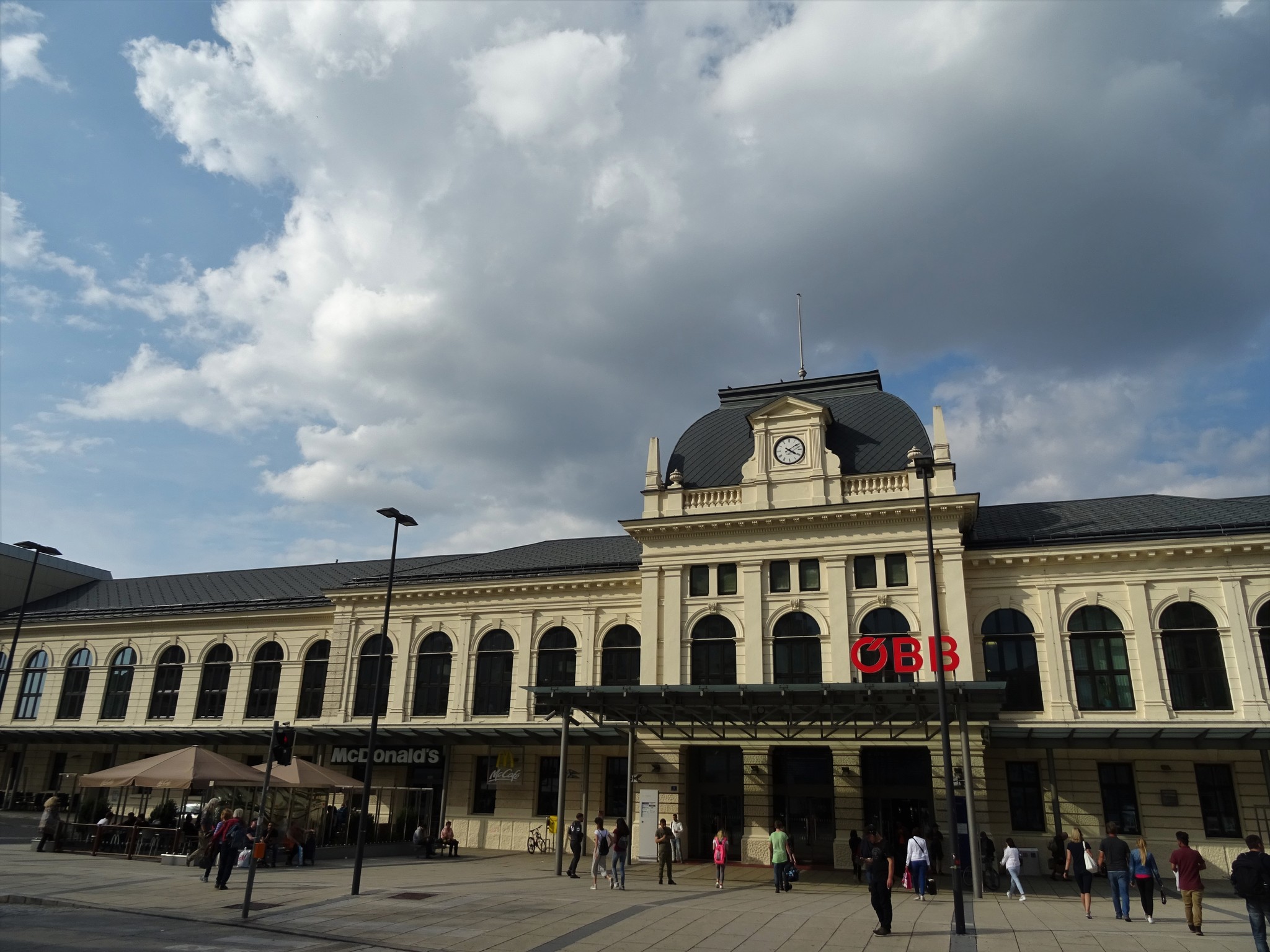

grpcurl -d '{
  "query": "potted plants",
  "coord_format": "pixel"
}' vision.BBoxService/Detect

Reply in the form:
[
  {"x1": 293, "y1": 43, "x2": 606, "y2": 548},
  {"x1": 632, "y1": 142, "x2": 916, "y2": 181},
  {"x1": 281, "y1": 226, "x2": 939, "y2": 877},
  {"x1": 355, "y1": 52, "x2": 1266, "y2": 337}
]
[{"x1": 78, "y1": 798, "x2": 111, "y2": 838}]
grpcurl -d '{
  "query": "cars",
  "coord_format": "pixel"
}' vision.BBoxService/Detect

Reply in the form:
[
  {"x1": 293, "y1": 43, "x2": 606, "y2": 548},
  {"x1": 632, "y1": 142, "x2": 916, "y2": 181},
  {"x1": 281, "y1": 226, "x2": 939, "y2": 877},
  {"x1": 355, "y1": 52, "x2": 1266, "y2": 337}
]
[{"x1": 173, "y1": 801, "x2": 208, "y2": 824}]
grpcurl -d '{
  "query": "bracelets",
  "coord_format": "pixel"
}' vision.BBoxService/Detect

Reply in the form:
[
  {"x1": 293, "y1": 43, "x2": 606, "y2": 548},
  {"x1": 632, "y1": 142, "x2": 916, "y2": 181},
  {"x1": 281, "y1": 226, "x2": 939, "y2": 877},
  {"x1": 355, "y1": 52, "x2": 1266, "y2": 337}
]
[{"x1": 1064, "y1": 869, "x2": 1068, "y2": 872}]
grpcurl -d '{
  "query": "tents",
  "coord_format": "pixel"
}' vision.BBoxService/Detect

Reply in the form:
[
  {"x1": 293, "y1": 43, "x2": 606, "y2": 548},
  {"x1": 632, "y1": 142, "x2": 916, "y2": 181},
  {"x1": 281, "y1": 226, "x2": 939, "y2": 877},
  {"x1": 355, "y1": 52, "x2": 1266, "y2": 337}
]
[{"x1": 71, "y1": 745, "x2": 365, "y2": 854}]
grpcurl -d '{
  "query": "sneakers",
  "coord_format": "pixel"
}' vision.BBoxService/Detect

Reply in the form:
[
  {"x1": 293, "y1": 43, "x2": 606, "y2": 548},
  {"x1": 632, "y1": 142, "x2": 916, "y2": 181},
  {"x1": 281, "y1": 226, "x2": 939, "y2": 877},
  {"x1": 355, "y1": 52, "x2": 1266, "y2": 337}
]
[
  {"x1": 719, "y1": 884, "x2": 723, "y2": 888},
  {"x1": 716, "y1": 882, "x2": 719, "y2": 887},
  {"x1": 872, "y1": 927, "x2": 891, "y2": 937},
  {"x1": 566, "y1": 860, "x2": 683, "y2": 890}
]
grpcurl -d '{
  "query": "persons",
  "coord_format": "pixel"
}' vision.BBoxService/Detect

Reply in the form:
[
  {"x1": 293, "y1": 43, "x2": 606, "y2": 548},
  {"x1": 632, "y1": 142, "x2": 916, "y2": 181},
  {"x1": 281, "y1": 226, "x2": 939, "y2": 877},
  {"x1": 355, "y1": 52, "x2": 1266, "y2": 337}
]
[
  {"x1": 1049, "y1": 832, "x2": 1069, "y2": 882},
  {"x1": 1063, "y1": 827, "x2": 1093, "y2": 919},
  {"x1": 566, "y1": 813, "x2": 584, "y2": 879},
  {"x1": 1000, "y1": 838, "x2": 1026, "y2": 901},
  {"x1": 1236, "y1": 834, "x2": 1270, "y2": 952},
  {"x1": 36, "y1": 792, "x2": 357, "y2": 883},
  {"x1": 209, "y1": 808, "x2": 247, "y2": 890},
  {"x1": 670, "y1": 813, "x2": 683, "y2": 863},
  {"x1": 1169, "y1": 831, "x2": 1207, "y2": 936},
  {"x1": 769, "y1": 820, "x2": 796, "y2": 893},
  {"x1": 713, "y1": 828, "x2": 729, "y2": 889},
  {"x1": 591, "y1": 817, "x2": 629, "y2": 890},
  {"x1": 860, "y1": 824, "x2": 894, "y2": 937},
  {"x1": 1129, "y1": 837, "x2": 1163, "y2": 923},
  {"x1": 849, "y1": 822, "x2": 996, "y2": 901},
  {"x1": 413, "y1": 823, "x2": 436, "y2": 859},
  {"x1": 441, "y1": 821, "x2": 461, "y2": 857},
  {"x1": 1098, "y1": 821, "x2": 1131, "y2": 922},
  {"x1": 655, "y1": 818, "x2": 677, "y2": 885}
]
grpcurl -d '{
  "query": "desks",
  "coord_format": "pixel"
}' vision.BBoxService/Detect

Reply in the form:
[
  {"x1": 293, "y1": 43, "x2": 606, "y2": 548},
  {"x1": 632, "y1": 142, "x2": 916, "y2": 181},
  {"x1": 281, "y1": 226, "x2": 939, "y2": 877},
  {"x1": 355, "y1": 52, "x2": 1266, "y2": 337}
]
[{"x1": 185, "y1": 834, "x2": 199, "y2": 853}]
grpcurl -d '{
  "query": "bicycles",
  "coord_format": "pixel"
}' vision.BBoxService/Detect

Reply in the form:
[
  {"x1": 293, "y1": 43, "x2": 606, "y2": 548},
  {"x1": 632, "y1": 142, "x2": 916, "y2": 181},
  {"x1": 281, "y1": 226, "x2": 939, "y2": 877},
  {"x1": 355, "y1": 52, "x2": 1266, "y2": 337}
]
[
  {"x1": 527, "y1": 825, "x2": 547, "y2": 854},
  {"x1": 963, "y1": 859, "x2": 1001, "y2": 891}
]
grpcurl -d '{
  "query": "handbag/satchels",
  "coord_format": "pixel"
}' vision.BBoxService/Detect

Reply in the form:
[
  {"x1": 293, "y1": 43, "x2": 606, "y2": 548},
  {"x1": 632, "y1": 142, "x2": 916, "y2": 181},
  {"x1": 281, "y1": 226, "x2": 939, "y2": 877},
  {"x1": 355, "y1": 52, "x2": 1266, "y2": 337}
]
[
  {"x1": 285, "y1": 848, "x2": 294, "y2": 858},
  {"x1": 926, "y1": 873, "x2": 938, "y2": 895},
  {"x1": 786, "y1": 864, "x2": 800, "y2": 882},
  {"x1": 1082, "y1": 838, "x2": 1098, "y2": 873},
  {"x1": 902, "y1": 868, "x2": 912, "y2": 889},
  {"x1": 1161, "y1": 887, "x2": 1166, "y2": 904},
  {"x1": 1019, "y1": 857, "x2": 1023, "y2": 866},
  {"x1": 1047, "y1": 839, "x2": 1058, "y2": 850},
  {"x1": 228, "y1": 820, "x2": 247, "y2": 848},
  {"x1": 1173, "y1": 870, "x2": 1181, "y2": 890},
  {"x1": 199, "y1": 844, "x2": 214, "y2": 868},
  {"x1": 237, "y1": 847, "x2": 251, "y2": 868}
]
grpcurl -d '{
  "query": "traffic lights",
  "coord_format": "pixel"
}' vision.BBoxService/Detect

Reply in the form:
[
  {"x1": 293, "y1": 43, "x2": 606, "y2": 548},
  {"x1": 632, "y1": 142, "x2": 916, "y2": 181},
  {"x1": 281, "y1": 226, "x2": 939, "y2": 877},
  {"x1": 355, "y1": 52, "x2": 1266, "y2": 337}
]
[{"x1": 272, "y1": 727, "x2": 297, "y2": 766}]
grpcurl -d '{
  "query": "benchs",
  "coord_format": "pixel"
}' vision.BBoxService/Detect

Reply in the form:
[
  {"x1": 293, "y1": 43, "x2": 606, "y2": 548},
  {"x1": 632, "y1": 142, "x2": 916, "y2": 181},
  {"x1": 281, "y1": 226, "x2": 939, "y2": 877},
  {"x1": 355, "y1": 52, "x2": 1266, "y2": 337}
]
[
  {"x1": 411, "y1": 844, "x2": 447, "y2": 859},
  {"x1": 238, "y1": 847, "x2": 315, "y2": 865}
]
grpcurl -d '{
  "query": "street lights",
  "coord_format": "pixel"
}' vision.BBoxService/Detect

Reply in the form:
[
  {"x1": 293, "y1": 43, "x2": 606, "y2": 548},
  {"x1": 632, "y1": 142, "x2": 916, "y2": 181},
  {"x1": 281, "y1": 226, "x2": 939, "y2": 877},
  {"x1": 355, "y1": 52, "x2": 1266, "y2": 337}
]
[
  {"x1": 349, "y1": 506, "x2": 418, "y2": 896},
  {"x1": 914, "y1": 452, "x2": 967, "y2": 937},
  {"x1": 0, "y1": 540, "x2": 64, "y2": 715}
]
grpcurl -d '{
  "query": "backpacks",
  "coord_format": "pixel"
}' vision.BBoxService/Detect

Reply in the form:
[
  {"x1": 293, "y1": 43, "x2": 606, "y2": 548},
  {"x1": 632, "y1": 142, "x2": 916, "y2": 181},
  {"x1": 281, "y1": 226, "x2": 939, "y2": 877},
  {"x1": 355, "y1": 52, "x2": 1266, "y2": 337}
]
[
  {"x1": 597, "y1": 828, "x2": 609, "y2": 855},
  {"x1": 714, "y1": 837, "x2": 726, "y2": 865},
  {"x1": 1233, "y1": 852, "x2": 1262, "y2": 896}
]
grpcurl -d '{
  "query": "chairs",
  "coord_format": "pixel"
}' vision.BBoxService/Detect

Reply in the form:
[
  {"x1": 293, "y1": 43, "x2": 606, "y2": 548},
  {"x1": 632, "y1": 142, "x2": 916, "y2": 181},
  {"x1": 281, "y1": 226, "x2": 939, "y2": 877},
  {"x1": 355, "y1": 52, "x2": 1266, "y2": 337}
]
[
  {"x1": 14, "y1": 792, "x2": 80, "y2": 813},
  {"x1": 141, "y1": 828, "x2": 186, "y2": 853}
]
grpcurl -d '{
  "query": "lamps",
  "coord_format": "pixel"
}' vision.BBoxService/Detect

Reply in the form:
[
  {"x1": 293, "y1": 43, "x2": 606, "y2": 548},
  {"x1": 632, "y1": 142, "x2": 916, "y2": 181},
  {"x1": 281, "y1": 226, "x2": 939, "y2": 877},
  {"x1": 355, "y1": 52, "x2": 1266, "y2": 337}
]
[
  {"x1": 954, "y1": 767, "x2": 962, "y2": 775},
  {"x1": 1161, "y1": 764, "x2": 1171, "y2": 771},
  {"x1": 841, "y1": 767, "x2": 850, "y2": 772},
  {"x1": 512, "y1": 758, "x2": 519, "y2": 762},
  {"x1": 651, "y1": 764, "x2": 661, "y2": 768},
  {"x1": 751, "y1": 766, "x2": 759, "y2": 770}
]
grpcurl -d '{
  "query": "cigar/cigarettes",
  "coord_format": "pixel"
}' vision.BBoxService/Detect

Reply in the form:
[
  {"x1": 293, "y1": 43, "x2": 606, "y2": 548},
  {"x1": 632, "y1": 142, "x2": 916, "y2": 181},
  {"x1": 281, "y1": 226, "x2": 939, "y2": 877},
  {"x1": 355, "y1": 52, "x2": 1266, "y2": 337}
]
[{"x1": 888, "y1": 888, "x2": 889, "y2": 890}]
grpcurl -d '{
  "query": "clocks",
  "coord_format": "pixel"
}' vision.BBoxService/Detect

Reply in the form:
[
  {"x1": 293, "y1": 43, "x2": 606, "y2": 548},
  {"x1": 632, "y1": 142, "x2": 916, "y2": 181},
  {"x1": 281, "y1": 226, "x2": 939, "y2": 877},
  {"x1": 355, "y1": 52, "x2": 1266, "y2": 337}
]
[{"x1": 775, "y1": 437, "x2": 804, "y2": 463}]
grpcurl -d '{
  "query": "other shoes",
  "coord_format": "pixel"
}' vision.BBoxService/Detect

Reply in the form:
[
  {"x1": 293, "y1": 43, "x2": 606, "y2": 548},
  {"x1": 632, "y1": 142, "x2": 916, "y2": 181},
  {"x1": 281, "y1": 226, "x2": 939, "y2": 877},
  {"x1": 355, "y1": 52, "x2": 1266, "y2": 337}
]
[
  {"x1": 772, "y1": 880, "x2": 792, "y2": 893},
  {"x1": 183, "y1": 857, "x2": 314, "y2": 890},
  {"x1": 920, "y1": 896, "x2": 925, "y2": 901},
  {"x1": 1004, "y1": 889, "x2": 1204, "y2": 937},
  {"x1": 913, "y1": 894, "x2": 920, "y2": 900},
  {"x1": 426, "y1": 851, "x2": 460, "y2": 860}
]
